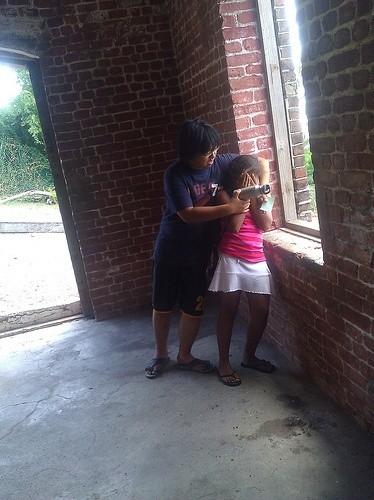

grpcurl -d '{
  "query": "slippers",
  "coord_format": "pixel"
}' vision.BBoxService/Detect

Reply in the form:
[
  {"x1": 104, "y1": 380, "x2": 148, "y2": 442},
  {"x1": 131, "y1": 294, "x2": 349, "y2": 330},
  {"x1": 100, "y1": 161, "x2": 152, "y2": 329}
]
[
  {"x1": 144, "y1": 355, "x2": 171, "y2": 377},
  {"x1": 176, "y1": 358, "x2": 216, "y2": 373},
  {"x1": 240, "y1": 359, "x2": 274, "y2": 372},
  {"x1": 217, "y1": 368, "x2": 241, "y2": 386}
]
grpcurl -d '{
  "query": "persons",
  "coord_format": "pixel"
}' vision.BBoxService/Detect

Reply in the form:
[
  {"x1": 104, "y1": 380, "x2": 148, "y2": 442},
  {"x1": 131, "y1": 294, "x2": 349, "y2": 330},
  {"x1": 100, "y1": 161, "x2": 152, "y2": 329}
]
[
  {"x1": 209, "y1": 155, "x2": 277, "y2": 386},
  {"x1": 144, "y1": 119, "x2": 271, "y2": 378}
]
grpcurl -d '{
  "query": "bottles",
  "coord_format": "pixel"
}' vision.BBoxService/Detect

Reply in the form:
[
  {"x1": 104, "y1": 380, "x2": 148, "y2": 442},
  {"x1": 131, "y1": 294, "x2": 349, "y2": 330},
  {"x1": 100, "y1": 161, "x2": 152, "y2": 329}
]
[{"x1": 238, "y1": 185, "x2": 270, "y2": 200}]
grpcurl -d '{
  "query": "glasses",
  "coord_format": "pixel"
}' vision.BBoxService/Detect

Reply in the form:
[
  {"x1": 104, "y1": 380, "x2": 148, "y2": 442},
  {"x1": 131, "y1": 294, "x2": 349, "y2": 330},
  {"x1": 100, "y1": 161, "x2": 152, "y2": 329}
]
[{"x1": 197, "y1": 146, "x2": 221, "y2": 158}]
